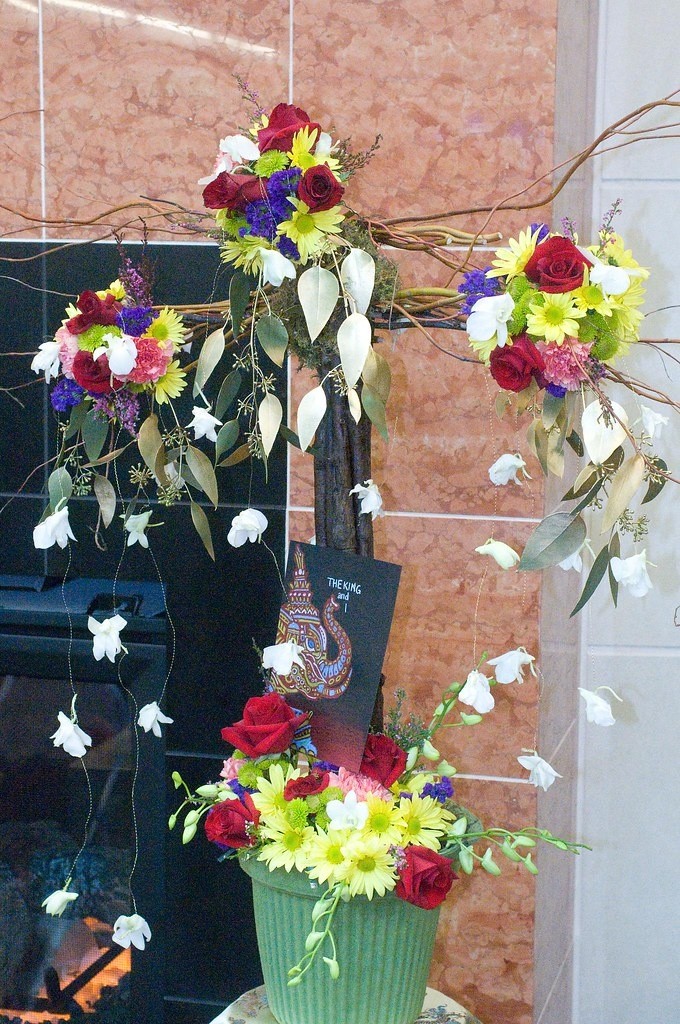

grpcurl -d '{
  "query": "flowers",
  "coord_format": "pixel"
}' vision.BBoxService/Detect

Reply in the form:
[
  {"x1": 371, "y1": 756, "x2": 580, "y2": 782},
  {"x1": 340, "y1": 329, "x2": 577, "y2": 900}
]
[{"x1": 0, "y1": 69, "x2": 680, "y2": 987}]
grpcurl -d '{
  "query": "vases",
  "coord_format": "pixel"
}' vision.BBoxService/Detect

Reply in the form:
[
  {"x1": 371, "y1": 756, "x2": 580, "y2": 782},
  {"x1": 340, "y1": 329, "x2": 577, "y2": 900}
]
[{"x1": 237, "y1": 848, "x2": 443, "y2": 1024}]
